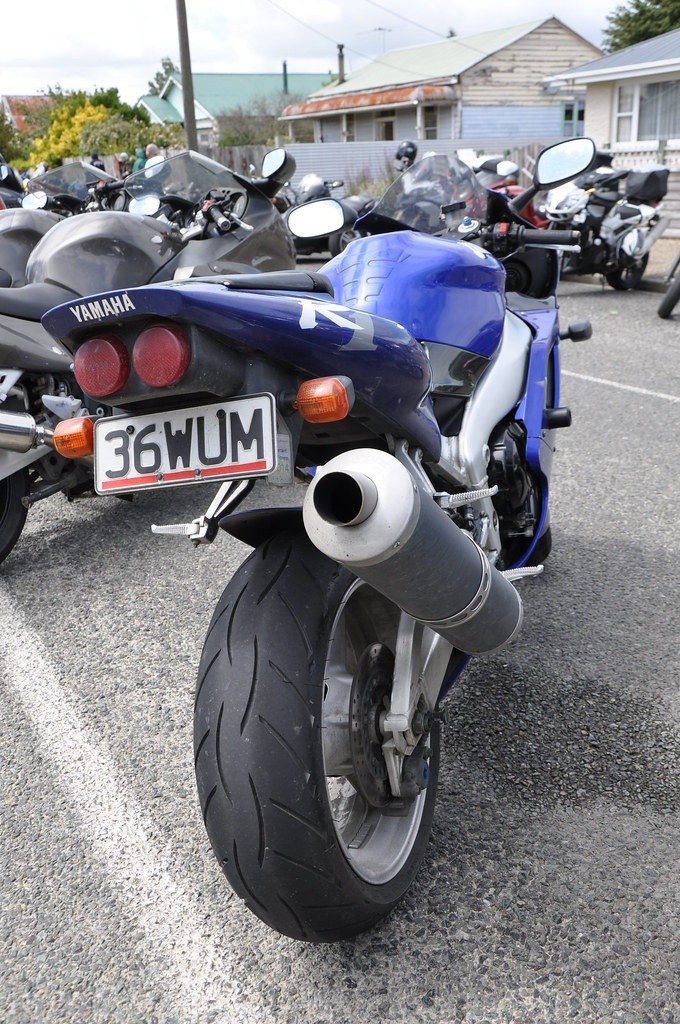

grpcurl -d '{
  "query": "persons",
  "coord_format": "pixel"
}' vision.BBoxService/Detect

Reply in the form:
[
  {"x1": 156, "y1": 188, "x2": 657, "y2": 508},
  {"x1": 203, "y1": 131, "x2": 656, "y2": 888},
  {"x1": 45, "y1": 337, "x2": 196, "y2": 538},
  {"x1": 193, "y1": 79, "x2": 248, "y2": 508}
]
[
  {"x1": 91, "y1": 154, "x2": 107, "y2": 174},
  {"x1": 131, "y1": 145, "x2": 149, "y2": 177},
  {"x1": 145, "y1": 145, "x2": 166, "y2": 181},
  {"x1": 115, "y1": 149, "x2": 136, "y2": 181},
  {"x1": 18, "y1": 161, "x2": 52, "y2": 193}
]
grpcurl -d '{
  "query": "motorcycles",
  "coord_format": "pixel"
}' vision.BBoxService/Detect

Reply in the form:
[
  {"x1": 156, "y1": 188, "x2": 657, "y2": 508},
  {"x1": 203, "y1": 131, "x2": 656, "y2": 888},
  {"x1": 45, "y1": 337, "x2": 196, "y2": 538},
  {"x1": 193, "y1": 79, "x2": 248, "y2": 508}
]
[{"x1": 0, "y1": 144, "x2": 679, "y2": 942}]
